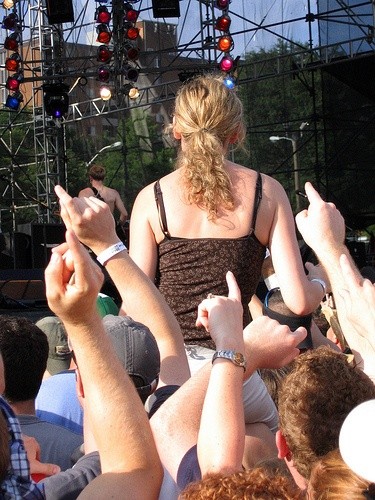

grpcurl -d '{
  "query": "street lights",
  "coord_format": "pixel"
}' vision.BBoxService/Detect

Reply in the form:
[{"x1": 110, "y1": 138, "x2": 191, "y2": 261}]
[
  {"x1": 86, "y1": 140, "x2": 124, "y2": 168},
  {"x1": 269, "y1": 134, "x2": 301, "y2": 211}
]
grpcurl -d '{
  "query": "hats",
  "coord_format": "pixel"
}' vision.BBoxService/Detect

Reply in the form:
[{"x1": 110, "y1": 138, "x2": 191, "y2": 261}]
[
  {"x1": 100, "y1": 314, "x2": 161, "y2": 397},
  {"x1": 95, "y1": 292, "x2": 118, "y2": 319},
  {"x1": 33, "y1": 315, "x2": 72, "y2": 375},
  {"x1": 263, "y1": 286, "x2": 313, "y2": 350}
]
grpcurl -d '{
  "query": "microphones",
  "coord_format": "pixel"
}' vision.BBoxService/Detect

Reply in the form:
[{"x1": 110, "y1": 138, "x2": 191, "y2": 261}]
[{"x1": 295, "y1": 190, "x2": 307, "y2": 197}]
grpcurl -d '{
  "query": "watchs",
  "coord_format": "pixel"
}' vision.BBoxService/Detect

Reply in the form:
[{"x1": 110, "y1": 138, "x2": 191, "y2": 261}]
[{"x1": 212, "y1": 350, "x2": 248, "y2": 373}]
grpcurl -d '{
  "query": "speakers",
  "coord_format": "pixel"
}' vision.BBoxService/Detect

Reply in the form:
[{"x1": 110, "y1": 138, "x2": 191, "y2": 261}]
[
  {"x1": 0, "y1": 231, "x2": 32, "y2": 270},
  {"x1": 16, "y1": 223, "x2": 67, "y2": 268}
]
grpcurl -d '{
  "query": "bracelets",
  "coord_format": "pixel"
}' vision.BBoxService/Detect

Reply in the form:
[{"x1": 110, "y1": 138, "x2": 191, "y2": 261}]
[
  {"x1": 96, "y1": 241, "x2": 128, "y2": 266},
  {"x1": 311, "y1": 278, "x2": 328, "y2": 296}
]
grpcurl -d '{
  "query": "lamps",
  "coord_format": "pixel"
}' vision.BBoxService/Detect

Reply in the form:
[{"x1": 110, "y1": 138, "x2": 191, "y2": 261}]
[
  {"x1": 124, "y1": 7, "x2": 139, "y2": 21},
  {"x1": 226, "y1": 76, "x2": 236, "y2": 86},
  {"x1": 5, "y1": 53, "x2": 21, "y2": 71},
  {"x1": 97, "y1": 45, "x2": 109, "y2": 62},
  {"x1": 96, "y1": 24, "x2": 112, "y2": 43},
  {"x1": 217, "y1": 35, "x2": 234, "y2": 53},
  {"x1": 152, "y1": 0, "x2": 180, "y2": 18},
  {"x1": 3, "y1": 0, "x2": 14, "y2": 10},
  {"x1": 3, "y1": 13, "x2": 18, "y2": 29},
  {"x1": 215, "y1": 0, "x2": 230, "y2": 10},
  {"x1": 96, "y1": 68, "x2": 111, "y2": 83},
  {"x1": 94, "y1": 6, "x2": 111, "y2": 24},
  {"x1": 124, "y1": 47, "x2": 138, "y2": 60},
  {"x1": 124, "y1": 27, "x2": 139, "y2": 40},
  {"x1": 5, "y1": 73, "x2": 24, "y2": 90},
  {"x1": 100, "y1": 85, "x2": 111, "y2": 100},
  {"x1": 46, "y1": 0, "x2": 74, "y2": 25},
  {"x1": 4, "y1": 33, "x2": 19, "y2": 51},
  {"x1": 123, "y1": 66, "x2": 139, "y2": 82},
  {"x1": 122, "y1": 84, "x2": 140, "y2": 99},
  {"x1": 42, "y1": 83, "x2": 70, "y2": 118},
  {"x1": 216, "y1": 14, "x2": 231, "y2": 32},
  {"x1": 220, "y1": 56, "x2": 233, "y2": 72},
  {"x1": 5, "y1": 91, "x2": 24, "y2": 110}
]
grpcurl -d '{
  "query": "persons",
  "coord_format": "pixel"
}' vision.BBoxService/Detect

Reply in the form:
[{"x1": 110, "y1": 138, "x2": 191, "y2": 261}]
[
  {"x1": 305, "y1": 447, "x2": 375, "y2": 500},
  {"x1": 78, "y1": 165, "x2": 128, "y2": 229},
  {"x1": 0, "y1": 348, "x2": 102, "y2": 500},
  {"x1": 0, "y1": 226, "x2": 374, "y2": 498},
  {"x1": 149, "y1": 316, "x2": 308, "y2": 490},
  {"x1": 195, "y1": 271, "x2": 375, "y2": 479},
  {"x1": 117, "y1": 75, "x2": 333, "y2": 436},
  {"x1": 34, "y1": 331, "x2": 83, "y2": 438},
  {"x1": 44, "y1": 228, "x2": 165, "y2": 500},
  {"x1": 336, "y1": 253, "x2": 374, "y2": 351},
  {"x1": 295, "y1": 182, "x2": 374, "y2": 384},
  {"x1": 53, "y1": 184, "x2": 191, "y2": 421},
  {"x1": 177, "y1": 467, "x2": 308, "y2": 500},
  {"x1": 0, "y1": 315, "x2": 84, "y2": 470},
  {"x1": 36, "y1": 316, "x2": 73, "y2": 380}
]
